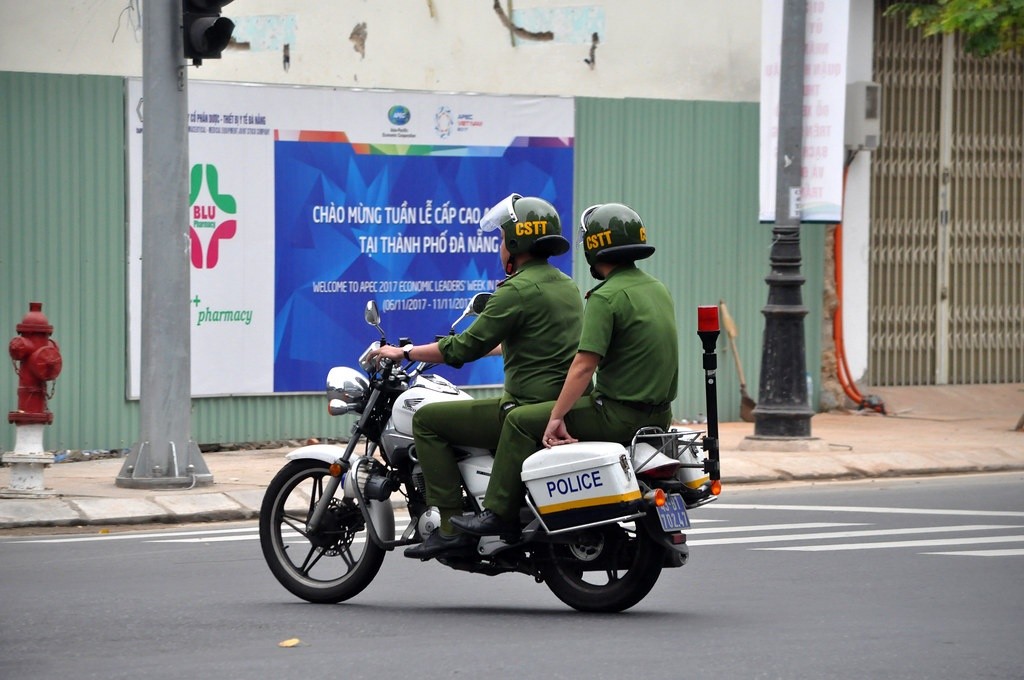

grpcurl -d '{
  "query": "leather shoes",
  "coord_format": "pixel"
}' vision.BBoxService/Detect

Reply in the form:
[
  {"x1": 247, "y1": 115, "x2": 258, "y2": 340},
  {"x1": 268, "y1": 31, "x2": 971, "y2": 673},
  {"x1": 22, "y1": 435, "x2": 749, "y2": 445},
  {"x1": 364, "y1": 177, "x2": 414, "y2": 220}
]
[
  {"x1": 452, "y1": 511, "x2": 520, "y2": 541},
  {"x1": 403, "y1": 526, "x2": 476, "y2": 557}
]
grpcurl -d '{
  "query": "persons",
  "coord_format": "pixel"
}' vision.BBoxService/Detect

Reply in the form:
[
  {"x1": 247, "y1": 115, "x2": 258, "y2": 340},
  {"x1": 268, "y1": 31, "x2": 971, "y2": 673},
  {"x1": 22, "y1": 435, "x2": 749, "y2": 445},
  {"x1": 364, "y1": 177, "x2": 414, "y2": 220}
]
[
  {"x1": 364, "y1": 192, "x2": 596, "y2": 559},
  {"x1": 447, "y1": 202, "x2": 679, "y2": 545}
]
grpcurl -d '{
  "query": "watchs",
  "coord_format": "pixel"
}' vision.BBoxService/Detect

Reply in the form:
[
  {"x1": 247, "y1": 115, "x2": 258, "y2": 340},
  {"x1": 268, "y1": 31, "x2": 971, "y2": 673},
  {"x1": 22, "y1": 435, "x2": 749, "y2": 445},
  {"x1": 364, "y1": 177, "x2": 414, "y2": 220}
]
[{"x1": 403, "y1": 343, "x2": 414, "y2": 362}]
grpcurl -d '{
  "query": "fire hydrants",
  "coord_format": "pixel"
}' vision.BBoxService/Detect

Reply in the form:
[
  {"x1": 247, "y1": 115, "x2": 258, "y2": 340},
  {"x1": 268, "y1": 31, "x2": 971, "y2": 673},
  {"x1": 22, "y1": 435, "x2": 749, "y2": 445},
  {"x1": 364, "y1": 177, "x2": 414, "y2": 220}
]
[{"x1": 2, "y1": 302, "x2": 61, "y2": 498}]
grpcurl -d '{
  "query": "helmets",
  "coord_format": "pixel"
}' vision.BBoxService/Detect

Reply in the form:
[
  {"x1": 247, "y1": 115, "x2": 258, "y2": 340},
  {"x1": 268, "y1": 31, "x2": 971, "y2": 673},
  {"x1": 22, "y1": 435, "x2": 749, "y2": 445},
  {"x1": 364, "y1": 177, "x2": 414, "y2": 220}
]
[
  {"x1": 577, "y1": 203, "x2": 655, "y2": 262},
  {"x1": 502, "y1": 197, "x2": 572, "y2": 256}
]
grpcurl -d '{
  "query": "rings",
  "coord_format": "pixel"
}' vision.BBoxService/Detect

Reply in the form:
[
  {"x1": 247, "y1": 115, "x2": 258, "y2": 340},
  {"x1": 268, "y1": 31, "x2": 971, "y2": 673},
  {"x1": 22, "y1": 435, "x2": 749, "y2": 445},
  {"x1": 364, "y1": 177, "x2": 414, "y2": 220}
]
[{"x1": 547, "y1": 438, "x2": 552, "y2": 444}]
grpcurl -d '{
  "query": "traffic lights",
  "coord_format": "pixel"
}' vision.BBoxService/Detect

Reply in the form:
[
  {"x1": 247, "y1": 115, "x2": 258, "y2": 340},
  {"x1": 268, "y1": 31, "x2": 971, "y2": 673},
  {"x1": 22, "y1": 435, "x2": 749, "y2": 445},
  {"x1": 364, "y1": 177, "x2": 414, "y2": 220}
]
[{"x1": 180, "y1": 1, "x2": 233, "y2": 66}]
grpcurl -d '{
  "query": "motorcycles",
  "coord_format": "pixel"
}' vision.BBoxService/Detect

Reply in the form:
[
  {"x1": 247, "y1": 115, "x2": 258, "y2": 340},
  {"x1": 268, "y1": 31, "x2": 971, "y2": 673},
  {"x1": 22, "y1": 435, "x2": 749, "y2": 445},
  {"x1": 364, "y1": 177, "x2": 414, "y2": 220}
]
[{"x1": 258, "y1": 291, "x2": 723, "y2": 615}]
